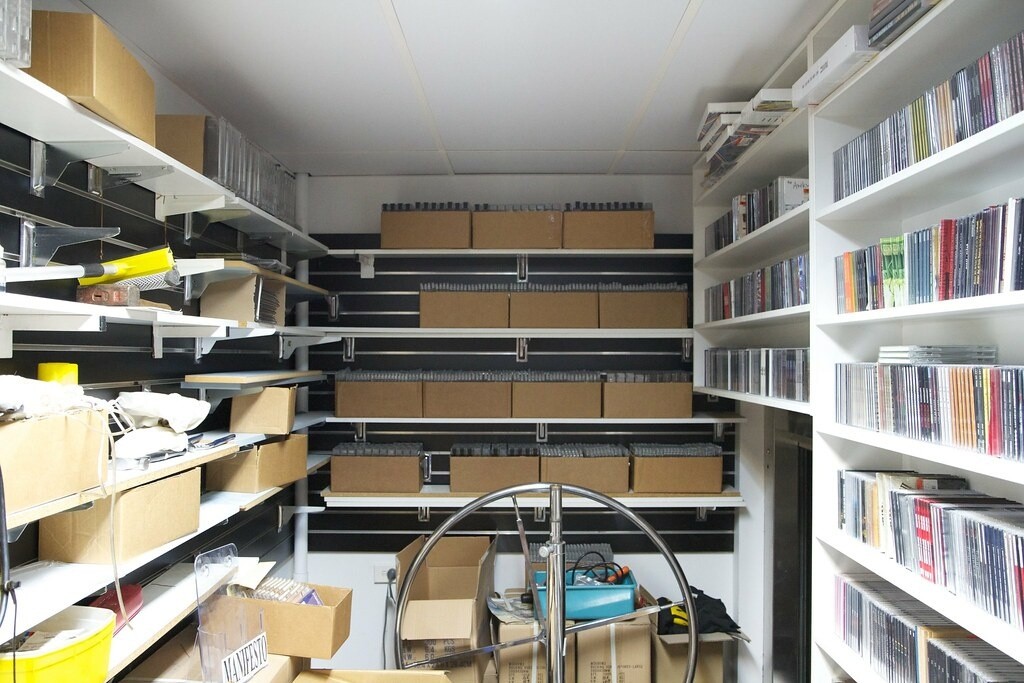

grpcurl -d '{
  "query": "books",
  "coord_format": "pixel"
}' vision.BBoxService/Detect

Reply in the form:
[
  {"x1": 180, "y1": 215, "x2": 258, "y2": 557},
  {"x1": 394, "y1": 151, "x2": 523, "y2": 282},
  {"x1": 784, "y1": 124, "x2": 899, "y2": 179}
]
[
  {"x1": 235, "y1": 577, "x2": 323, "y2": 605},
  {"x1": 325, "y1": 201, "x2": 722, "y2": 563},
  {"x1": 696, "y1": 1, "x2": 1024, "y2": 683},
  {"x1": 205, "y1": 117, "x2": 296, "y2": 224},
  {"x1": 196, "y1": 253, "x2": 292, "y2": 326}
]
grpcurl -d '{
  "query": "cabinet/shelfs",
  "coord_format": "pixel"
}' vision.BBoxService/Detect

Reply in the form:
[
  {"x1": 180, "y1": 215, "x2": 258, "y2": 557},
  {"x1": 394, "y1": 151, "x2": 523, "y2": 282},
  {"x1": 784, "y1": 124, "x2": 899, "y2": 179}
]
[
  {"x1": 693, "y1": 0, "x2": 1024, "y2": 683},
  {"x1": 0, "y1": 56, "x2": 329, "y2": 683},
  {"x1": 320, "y1": 248, "x2": 746, "y2": 535}
]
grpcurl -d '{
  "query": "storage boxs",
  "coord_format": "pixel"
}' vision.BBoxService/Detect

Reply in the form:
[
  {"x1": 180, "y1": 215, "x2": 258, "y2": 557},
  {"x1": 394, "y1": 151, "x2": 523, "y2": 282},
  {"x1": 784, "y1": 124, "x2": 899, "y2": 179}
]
[
  {"x1": 0, "y1": 10, "x2": 751, "y2": 683},
  {"x1": 791, "y1": 25, "x2": 880, "y2": 108}
]
